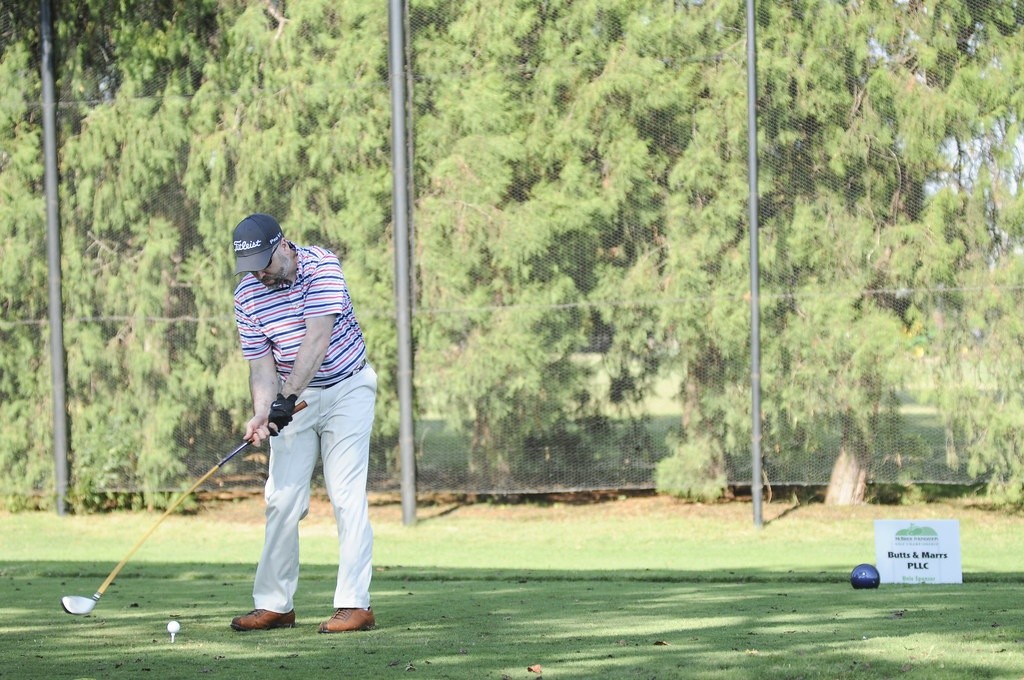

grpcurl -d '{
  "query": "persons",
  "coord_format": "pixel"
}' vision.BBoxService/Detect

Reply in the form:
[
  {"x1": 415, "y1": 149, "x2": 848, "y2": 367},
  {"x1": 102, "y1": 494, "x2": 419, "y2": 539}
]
[{"x1": 231, "y1": 214, "x2": 378, "y2": 632}]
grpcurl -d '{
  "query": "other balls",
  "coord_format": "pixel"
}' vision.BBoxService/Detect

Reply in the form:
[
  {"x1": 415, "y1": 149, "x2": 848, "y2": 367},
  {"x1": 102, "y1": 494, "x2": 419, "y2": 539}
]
[{"x1": 850, "y1": 563, "x2": 880, "y2": 589}]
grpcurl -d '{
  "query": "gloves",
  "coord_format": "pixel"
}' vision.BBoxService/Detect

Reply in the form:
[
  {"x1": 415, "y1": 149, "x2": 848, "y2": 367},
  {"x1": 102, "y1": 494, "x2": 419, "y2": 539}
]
[{"x1": 267, "y1": 393, "x2": 298, "y2": 437}]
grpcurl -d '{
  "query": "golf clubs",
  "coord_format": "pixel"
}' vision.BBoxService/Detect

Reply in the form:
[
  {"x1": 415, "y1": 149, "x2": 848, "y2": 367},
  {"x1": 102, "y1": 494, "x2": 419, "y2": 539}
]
[{"x1": 60, "y1": 399, "x2": 310, "y2": 617}]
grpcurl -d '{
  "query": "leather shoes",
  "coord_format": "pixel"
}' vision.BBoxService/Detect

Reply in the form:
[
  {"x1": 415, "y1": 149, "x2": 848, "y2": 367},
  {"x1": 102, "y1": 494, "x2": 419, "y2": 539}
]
[
  {"x1": 318, "y1": 607, "x2": 376, "y2": 633},
  {"x1": 229, "y1": 608, "x2": 296, "y2": 631}
]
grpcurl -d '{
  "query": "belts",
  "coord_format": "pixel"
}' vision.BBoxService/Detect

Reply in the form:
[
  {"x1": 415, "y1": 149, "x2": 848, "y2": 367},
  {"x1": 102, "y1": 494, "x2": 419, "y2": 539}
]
[{"x1": 304, "y1": 357, "x2": 367, "y2": 388}]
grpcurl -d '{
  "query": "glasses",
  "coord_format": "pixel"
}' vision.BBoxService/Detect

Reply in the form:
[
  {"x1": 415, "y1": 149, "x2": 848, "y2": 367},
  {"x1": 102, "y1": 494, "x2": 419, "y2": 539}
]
[{"x1": 247, "y1": 245, "x2": 280, "y2": 272}]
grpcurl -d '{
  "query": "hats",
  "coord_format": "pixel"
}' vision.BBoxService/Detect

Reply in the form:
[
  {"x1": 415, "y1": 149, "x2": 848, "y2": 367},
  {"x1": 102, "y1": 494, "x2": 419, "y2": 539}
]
[{"x1": 232, "y1": 213, "x2": 284, "y2": 276}]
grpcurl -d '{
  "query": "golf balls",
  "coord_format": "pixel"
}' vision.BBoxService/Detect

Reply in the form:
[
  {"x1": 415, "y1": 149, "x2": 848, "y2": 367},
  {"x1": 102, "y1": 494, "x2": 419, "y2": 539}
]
[{"x1": 167, "y1": 621, "x2": 181, "y2": 633}]
